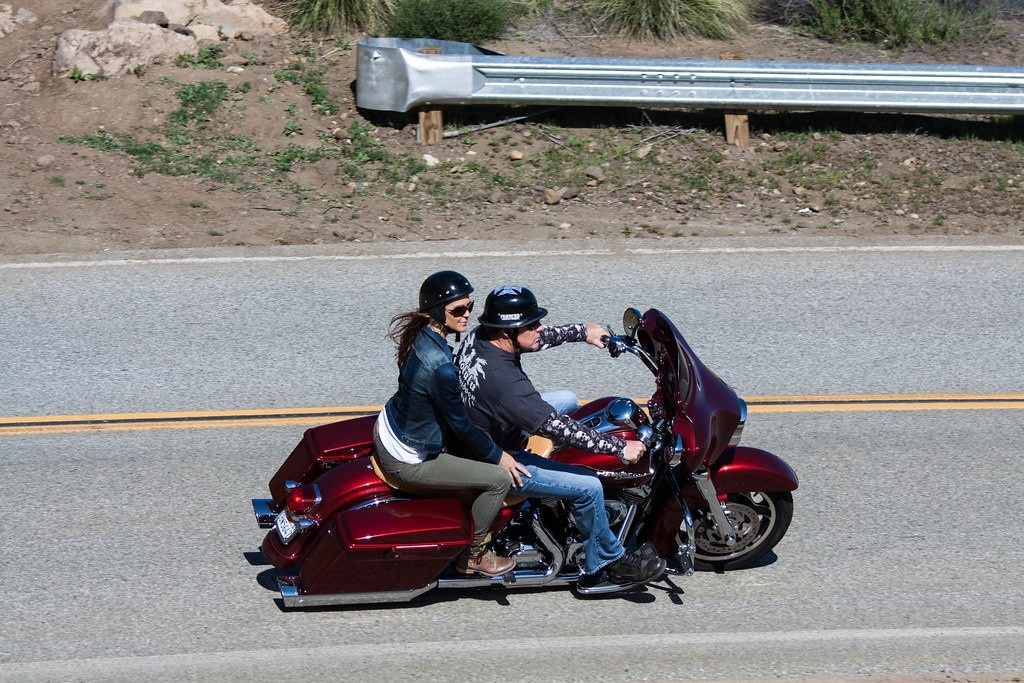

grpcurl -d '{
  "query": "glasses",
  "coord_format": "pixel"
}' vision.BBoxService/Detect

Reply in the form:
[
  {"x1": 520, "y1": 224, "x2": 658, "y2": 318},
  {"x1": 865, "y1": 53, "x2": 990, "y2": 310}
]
[
  {"x1": 530, "y1": 319, "x2": 541, "y2": 329},
  {"x1": 445, "y1": 301, "x2": 475, "y2": 317}
]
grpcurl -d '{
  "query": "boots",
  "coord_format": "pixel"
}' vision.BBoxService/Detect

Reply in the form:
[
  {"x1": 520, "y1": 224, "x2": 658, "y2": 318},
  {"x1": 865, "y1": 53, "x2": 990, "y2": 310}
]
[{"x1": 456, "y1": 534, "x2": 516, "y2": 575}]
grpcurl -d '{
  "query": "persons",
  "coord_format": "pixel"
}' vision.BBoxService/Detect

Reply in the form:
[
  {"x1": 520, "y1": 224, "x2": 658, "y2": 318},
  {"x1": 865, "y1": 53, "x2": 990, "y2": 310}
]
[
  {"x1": 371, "y1": 269, "x2": 533, "y2": 577},
  {"x1": 450, "y1": 283, "x2": 666, "y2": 585}
]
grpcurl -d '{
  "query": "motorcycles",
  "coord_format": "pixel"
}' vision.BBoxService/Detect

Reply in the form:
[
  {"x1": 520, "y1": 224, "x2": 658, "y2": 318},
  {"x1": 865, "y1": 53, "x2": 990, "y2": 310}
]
[{"x1": 251, "y1": 306, "x2": 800, "y2": 609}]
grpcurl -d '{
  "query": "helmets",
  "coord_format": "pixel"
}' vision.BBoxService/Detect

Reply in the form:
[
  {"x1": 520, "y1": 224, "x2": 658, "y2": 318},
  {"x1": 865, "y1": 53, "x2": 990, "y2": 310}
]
[
  {"x1": 478, "y1": 286, "x2": 548, "y2": 329},
  {"x1": 419, "y1": 270, "x2": 474, "y2": 311}
]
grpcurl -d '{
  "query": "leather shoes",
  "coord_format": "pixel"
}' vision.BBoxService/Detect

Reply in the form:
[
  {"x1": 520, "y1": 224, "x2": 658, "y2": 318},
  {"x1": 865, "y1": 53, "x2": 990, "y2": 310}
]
[{"x1": 607, "y1": 550, "x2": 666, "y2": 585}]
